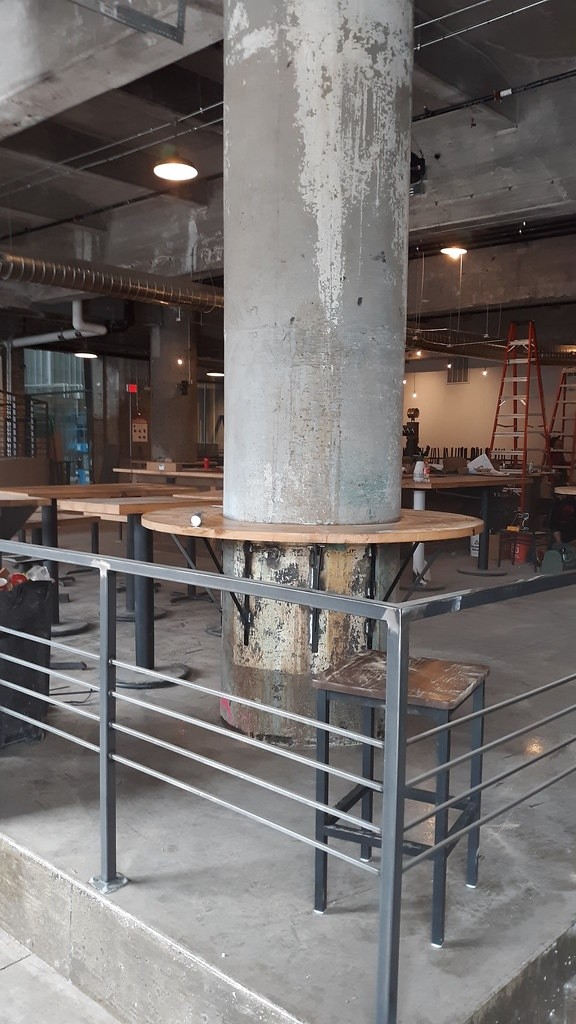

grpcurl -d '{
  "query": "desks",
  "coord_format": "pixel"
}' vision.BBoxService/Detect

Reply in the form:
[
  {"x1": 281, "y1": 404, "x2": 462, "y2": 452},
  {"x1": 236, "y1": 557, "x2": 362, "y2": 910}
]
[{"x1": 1, "y1": 469, "x2": 576, "y2": 745}]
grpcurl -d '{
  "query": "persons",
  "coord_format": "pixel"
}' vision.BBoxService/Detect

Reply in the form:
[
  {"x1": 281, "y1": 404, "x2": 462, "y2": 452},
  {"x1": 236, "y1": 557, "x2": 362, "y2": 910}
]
[
  {"x1": 550, "y1": 495, "x2": 576, "y2": 543},
  {"x1": 545, "y1": 436, "x2": 570, "y2": 486}
]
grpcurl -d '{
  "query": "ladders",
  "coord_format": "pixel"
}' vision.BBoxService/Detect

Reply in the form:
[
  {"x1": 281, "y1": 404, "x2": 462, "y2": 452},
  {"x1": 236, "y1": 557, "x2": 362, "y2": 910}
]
[
  {"x1": 479, "y1": 319, "x2": 557, "y2": 525},
  {"x1": 533, "y1": 364, "x2": 576, "y2": 529}
]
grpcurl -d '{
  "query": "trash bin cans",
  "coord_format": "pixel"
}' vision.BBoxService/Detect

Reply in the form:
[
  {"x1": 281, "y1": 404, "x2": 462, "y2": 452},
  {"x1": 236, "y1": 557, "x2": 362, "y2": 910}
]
[
  {"x1": 470, "y1": 528, "x2": 491, "y2": 558},
  {"x1": 0, "y1": 580, "x2": 58, "y2": 749}
]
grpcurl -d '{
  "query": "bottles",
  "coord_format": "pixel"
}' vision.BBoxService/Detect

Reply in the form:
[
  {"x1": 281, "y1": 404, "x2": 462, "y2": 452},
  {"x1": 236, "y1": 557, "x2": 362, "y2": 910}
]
[
  {"x1": 529, "y1": 460, "x2": 534, "y2": 474},
  {"x1": 204, "y1": 459, "x2": 209, "y2": 471}
]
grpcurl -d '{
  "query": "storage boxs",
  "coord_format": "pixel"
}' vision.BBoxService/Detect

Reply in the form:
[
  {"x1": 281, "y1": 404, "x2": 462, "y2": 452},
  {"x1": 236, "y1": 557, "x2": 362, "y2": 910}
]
[{"x1": 489, "y1": 531, "x2": 512, "y2": 559}]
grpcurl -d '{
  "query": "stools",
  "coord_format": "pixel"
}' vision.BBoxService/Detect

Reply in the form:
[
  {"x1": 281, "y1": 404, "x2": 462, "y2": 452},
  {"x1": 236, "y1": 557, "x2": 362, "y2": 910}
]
[{"x1": 311, "y1": 650, "x2": 489, "y2": 946}]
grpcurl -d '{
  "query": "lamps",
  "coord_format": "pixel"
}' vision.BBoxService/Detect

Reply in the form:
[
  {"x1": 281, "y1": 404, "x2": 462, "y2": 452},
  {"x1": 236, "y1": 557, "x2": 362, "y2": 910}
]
[
  {"x1": 440, "y1": 244, "x2": 467, "y2": 258},
  {"x1": 153, "y1": 138, "x2": 198, "y2": 181}
]
